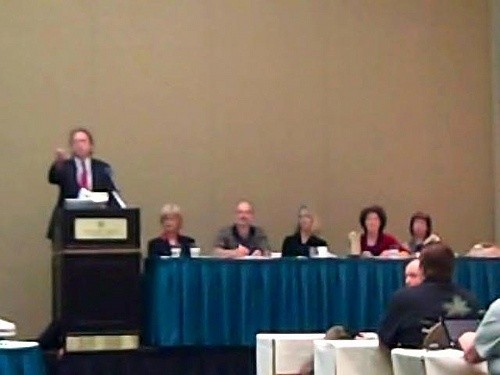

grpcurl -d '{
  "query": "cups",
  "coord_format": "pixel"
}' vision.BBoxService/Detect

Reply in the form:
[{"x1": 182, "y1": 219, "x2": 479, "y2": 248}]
[
  {"x1": 190, "y1": 248, "x2": 200, "y2": 257},
  {"x1": 317, "y1": 246, "x2": 327, "y2": 256}
]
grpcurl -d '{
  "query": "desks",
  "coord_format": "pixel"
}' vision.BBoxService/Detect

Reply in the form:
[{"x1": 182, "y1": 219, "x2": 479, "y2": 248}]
[
  {"x1": 146, "y1": 257, "x2": 500, "y2": 345},
  {"x1": 0, "y1": 339, "x2": 43, "y2": 374}
]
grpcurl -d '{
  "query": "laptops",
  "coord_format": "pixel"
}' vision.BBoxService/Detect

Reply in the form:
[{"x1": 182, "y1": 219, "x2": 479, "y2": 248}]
[{"x1": 442, "y1": 316, "x2": 482, "y2": 350}]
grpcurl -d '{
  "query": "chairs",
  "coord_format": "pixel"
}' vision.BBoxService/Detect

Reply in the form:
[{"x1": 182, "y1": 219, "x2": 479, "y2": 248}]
[{"x1": 249, "y1": 332, "x2": 489, "y2": 375}]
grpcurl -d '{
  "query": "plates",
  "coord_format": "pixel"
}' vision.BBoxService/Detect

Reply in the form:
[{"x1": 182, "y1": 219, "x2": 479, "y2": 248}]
[{"x1": 0, "y1": 340, "x2": 40, "y2": 350}]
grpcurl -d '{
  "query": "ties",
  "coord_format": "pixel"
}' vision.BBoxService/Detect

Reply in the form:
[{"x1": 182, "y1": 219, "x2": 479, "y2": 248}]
[{"x1": 81, "y1": 159, "x2": 88, "y2": 189}]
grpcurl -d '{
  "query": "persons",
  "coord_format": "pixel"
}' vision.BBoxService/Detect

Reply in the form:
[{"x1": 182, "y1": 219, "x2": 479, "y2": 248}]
[
  {"x1": 47, "y1": 128, "x2": 116, "y2": 244},
  {"x1": 379, "y1": 255, "x2": 478, "y2": 348},
  {"x1": 282, "y1": 206, "x2": 328, "y2": 258},
  {"x1": 348, "y1": 206, "x2": 410, "y2": 259},
  {"x1": 212, "y1": 199, "x2": 272, "y2": 260},
  {"x1": 464, "y1": 295, "x2": 500, "y2": 375},
  {"x1": 407, "y1": 212, "x2": 443, "y2": 259},
  {"x1": 147, "y1": 204, "x2": 196, "y2": 259}
]
